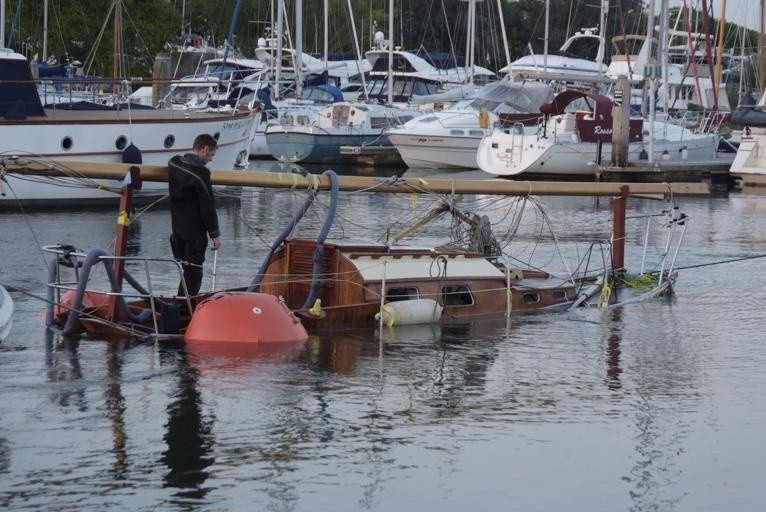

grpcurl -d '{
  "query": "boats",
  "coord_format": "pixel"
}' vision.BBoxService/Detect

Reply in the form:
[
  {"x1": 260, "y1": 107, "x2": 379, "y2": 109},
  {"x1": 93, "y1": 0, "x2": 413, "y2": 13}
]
[{"x1": 0, "y1": 154, "x2": 712, "y2": 343}]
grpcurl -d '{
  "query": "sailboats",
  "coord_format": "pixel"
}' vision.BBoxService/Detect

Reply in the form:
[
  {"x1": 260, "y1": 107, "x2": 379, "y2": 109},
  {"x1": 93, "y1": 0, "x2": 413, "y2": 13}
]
[{"x1": 0, "y1": 1, "x2": 766, "y2": 210}]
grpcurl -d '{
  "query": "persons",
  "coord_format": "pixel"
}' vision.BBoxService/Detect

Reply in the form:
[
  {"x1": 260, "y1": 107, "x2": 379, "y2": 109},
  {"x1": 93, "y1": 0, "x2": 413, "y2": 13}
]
[
  {"x1": 165, "y1": 131, "x2": 221, "y2": 291},
  {"x1": 155, "y1": 353, "x2": 215, "y2": 506}
]
